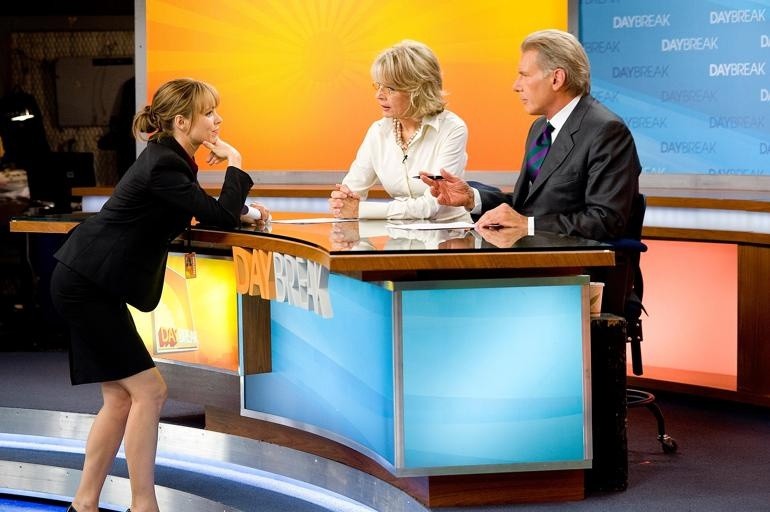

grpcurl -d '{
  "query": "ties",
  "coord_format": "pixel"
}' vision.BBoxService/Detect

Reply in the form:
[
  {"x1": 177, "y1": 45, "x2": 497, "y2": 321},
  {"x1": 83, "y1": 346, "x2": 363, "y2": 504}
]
[{"x1": 526, "y1": 121, "x2": 555, "y2": 185}]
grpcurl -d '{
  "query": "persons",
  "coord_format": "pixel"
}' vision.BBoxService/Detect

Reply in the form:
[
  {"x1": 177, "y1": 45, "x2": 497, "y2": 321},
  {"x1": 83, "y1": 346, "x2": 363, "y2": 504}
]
[
  {"x1": 329, "y1": 217, "x2": 480, "y2": 253},
  {"x1": 328, "y1": 39, "x2": 476, "y2": 224},
  {"x1": 438, "y1": 223, "x2": 613, "y2": 250},
  {"x1": 48, "y1": 77, "x2": 274, "y2": 512},
  {"x1": 417, "y1": 27, "x2": 643, "y2": 497}
]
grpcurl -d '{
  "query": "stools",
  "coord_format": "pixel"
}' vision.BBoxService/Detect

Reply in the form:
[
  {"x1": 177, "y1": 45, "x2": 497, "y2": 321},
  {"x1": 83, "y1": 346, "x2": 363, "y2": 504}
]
[{"x1": 626, "y1": 194, "x2": 677, "y2": 460}]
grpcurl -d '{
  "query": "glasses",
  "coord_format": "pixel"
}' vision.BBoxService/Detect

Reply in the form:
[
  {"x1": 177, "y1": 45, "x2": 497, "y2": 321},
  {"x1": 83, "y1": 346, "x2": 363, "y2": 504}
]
[{"x1": 372, "y1": 81, "x2": 399, "y2": 96}]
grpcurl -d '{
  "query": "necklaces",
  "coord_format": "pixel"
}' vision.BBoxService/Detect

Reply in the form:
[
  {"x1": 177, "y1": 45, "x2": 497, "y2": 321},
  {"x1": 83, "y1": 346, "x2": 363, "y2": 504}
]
[{"x1": 393, "y1": 116, "x2": 423, "y2": 152}]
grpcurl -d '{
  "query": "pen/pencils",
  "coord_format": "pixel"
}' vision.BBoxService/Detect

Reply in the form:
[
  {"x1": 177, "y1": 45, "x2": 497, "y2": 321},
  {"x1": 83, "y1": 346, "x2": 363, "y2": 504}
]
[{"x1": 412, "y1": 176, "x2": 444, "y2": 179}]
